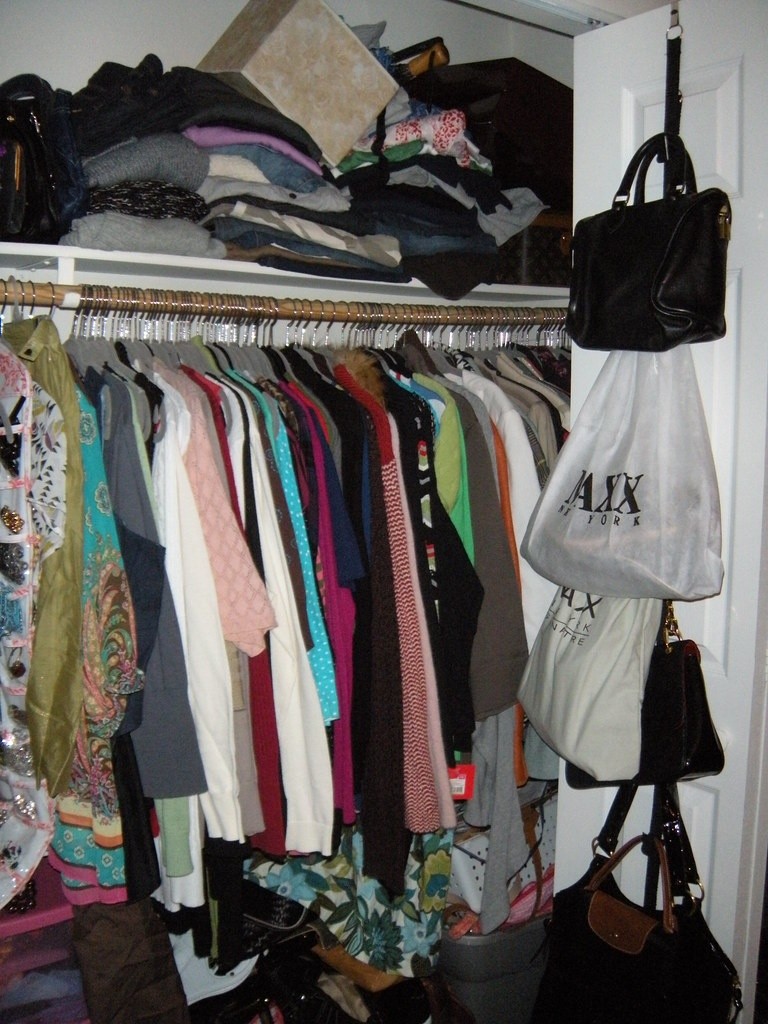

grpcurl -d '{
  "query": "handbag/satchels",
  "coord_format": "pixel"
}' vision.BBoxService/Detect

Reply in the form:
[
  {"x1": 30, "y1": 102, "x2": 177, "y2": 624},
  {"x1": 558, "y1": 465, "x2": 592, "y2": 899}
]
[
  {"x1": 0, "y1": 73, "x2": 88, "y2": 245},
  {"x1": 565, "y1": 599, "x2": 725, "y2": 789},
  {"x1": 565, "y1": 132, "x2": 732, "y2": 353},
  {"x1": 439, "y1": 903, "x2": 550, "y2": 1024},
  {"x1": 516, "y1": 582, "x2": 663, "y2": 781},
  {"x1": 527, "y1": 782, "x2": 744, "y2": 1024},
  {"x1": 520, "y1": 345, "x2": 725, "y2": 600}
]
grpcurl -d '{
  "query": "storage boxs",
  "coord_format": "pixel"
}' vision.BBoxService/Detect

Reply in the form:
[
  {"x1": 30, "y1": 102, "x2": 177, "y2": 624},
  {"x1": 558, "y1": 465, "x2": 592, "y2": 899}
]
[
  {"x1": 0, "y1": 855, "x2": 90, "y2": 1024},
  {"x1": 196, "y1": 0, "x2": 399, "y2": 169}
]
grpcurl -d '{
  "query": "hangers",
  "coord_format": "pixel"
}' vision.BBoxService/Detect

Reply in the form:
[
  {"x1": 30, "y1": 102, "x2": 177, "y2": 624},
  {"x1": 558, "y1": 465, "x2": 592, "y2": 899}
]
[{"x1": 0, "y1": 275, "x2": 572, "y2": 444}]
